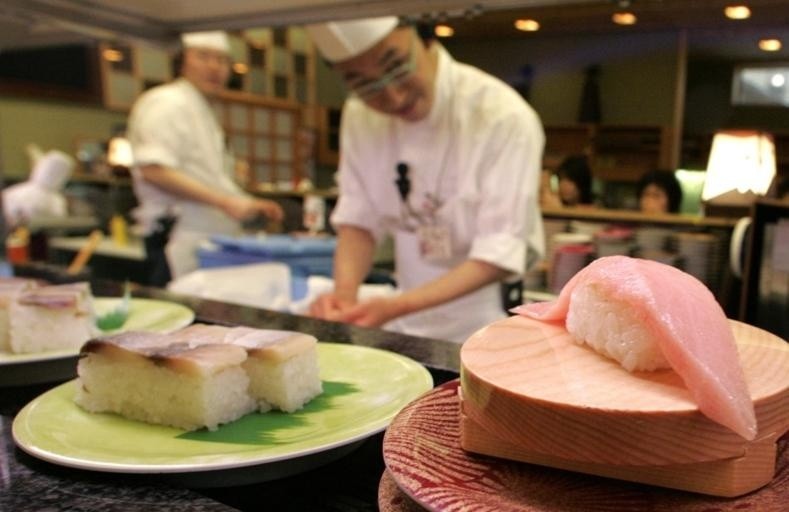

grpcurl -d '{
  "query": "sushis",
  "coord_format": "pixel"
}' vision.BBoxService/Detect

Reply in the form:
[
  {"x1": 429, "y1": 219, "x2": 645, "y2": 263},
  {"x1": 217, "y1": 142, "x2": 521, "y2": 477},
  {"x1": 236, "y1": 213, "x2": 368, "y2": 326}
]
[
  {"x1": 73, "y1": 323, "x2": 322, "y2": 432},
  {"x1": 508, "y1": 254, "x2": 757, "y2": 441},
  {"x1": 0, "y1": 276, "x2": 103, "y2": 358}
]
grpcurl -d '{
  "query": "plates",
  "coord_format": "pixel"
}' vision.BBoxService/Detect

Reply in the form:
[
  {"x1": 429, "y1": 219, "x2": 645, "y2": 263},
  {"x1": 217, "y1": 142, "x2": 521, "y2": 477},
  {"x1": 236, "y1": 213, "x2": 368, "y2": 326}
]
[
  {"x1": 0, "y1": 296, "x2": 196, "y2": 386},
  {"x1": 11, "y1": 342, "x2": 434, "y2": 473},
  {"x1": 383, "y1": 376, "x2": 789, "y2": 512}
]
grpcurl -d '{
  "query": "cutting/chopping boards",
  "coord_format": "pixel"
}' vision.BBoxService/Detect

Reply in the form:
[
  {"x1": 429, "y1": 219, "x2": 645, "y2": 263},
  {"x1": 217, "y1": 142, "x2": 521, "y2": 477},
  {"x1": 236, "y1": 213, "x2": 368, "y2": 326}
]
[{"x1": 458, "y1": 316, "x2": 789, "y2": 499}]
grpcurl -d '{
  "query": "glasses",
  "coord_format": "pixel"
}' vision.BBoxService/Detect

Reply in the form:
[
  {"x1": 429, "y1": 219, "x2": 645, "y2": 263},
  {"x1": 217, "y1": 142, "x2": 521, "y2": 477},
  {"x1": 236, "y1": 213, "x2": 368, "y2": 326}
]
[{"x1": 353, "y1": 29, "x2": 419, "y2": 101}]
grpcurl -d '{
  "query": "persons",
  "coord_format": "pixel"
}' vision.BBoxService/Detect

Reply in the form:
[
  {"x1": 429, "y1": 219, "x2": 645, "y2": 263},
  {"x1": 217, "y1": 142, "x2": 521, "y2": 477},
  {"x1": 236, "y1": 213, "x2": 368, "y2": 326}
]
[
  {"x1": 634, "y1": 172, "x2": 682, "y2": 213},
  {"x1": 124, "y1": 26, "x2": 290, "y2": 288},
  {"x1": 306, "y1": 13, "x2": 551, "y2": 347},
  {"x1": 541, "y1": 155, "x2": 610, "y2": 260},
  {"x1": 1, "y1": 148, "x2": 79, "y2": 228}
]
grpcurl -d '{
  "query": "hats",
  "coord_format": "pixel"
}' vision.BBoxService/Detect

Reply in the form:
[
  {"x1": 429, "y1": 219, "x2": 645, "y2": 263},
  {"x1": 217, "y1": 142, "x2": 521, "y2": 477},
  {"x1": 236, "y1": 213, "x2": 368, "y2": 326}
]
[
  {"x1": 182, "y1": 30, "x2": 230, "y2": 52},
  {"x1": 35, "y1": 151, "x2": 74, "y2": 189},
  {"x1": 308, "y1": 16, "x2": 399, "y2": 63}
]
